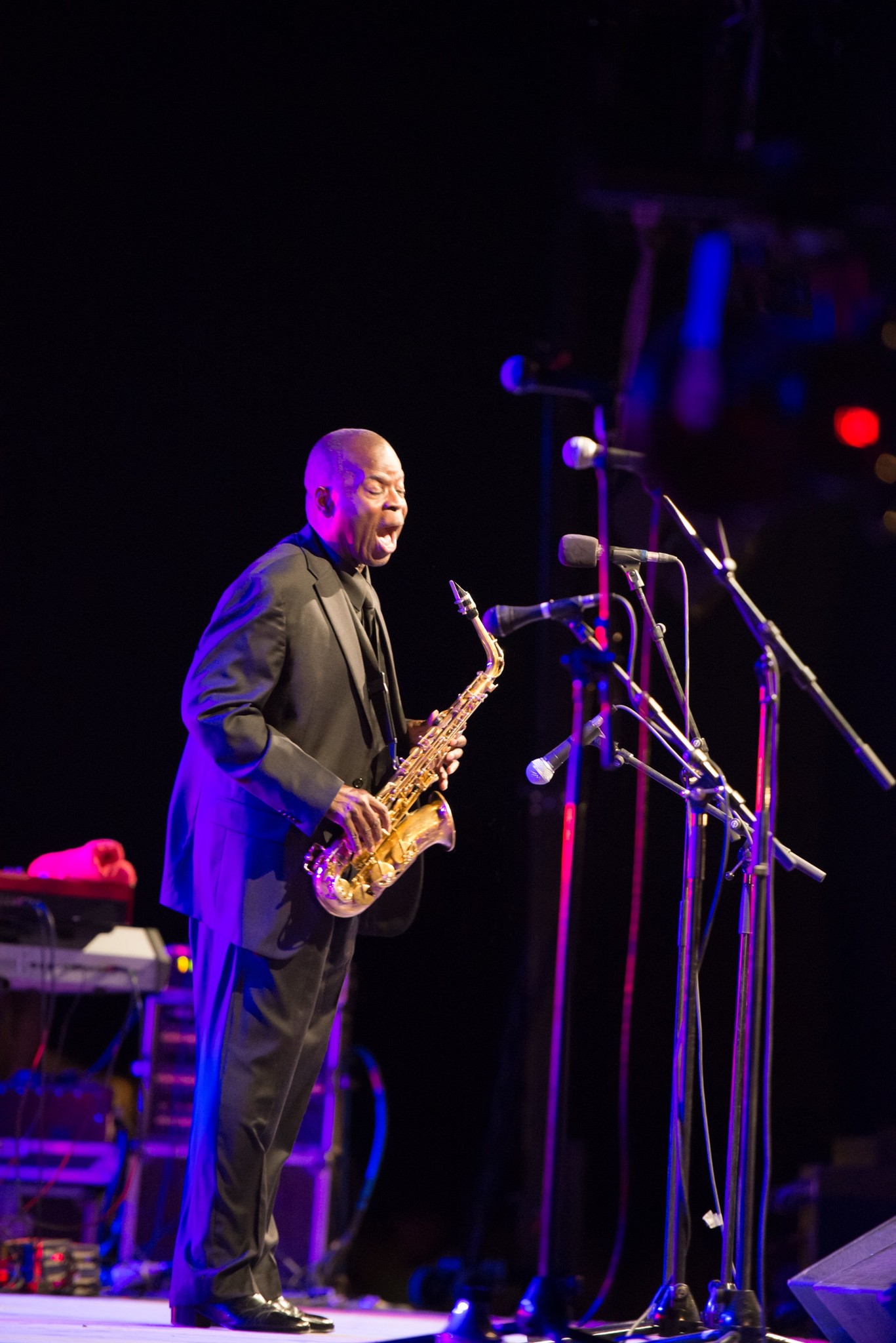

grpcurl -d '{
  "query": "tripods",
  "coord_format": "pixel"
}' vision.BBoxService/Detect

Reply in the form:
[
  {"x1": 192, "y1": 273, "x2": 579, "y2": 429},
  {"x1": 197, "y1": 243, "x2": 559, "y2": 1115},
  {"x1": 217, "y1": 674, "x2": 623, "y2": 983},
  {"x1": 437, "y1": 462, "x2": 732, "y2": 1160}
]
[{"x1": 377, "y1": 393, "x2": 896, "y2": 1341}]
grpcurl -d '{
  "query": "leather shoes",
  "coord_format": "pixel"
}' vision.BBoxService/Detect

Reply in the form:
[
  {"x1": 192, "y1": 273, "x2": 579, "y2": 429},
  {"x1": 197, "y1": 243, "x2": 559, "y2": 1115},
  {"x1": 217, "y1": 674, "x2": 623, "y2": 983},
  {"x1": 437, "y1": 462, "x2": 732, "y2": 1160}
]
[
  {"x1": 268, "y1": 1295, "x2": 335, "y2": 1333},
  {"x1": 170, "y1": 1295, "x2": 310, "y2": 1334}
]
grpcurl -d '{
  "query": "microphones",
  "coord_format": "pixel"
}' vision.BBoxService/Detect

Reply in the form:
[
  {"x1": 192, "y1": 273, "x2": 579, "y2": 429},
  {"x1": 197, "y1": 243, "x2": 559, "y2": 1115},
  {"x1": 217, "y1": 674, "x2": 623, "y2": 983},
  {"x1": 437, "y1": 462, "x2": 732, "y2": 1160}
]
[
  {"x1": 558, "y1": 534, "x2": 677, "y2": 567},
  {"x1": 563, "y1": 436, "x2": 650, "y2": 473},
  {"x1": 501, "y1": 355, "x2": 617, "y2": 398},
  {"x1": 526, "y1": 704, "x2": 617, "y2": 785},
  {"x1": 482, "y1": 592, "x2": 615, "y2": 637}
]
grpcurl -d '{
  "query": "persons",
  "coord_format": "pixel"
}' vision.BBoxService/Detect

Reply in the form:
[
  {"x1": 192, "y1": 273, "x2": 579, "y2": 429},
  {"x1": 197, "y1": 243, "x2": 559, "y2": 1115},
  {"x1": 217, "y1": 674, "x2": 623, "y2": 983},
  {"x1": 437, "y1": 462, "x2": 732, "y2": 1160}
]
[{"x1": 160, "y1": 427, "x2": 467, "y2": 1335}]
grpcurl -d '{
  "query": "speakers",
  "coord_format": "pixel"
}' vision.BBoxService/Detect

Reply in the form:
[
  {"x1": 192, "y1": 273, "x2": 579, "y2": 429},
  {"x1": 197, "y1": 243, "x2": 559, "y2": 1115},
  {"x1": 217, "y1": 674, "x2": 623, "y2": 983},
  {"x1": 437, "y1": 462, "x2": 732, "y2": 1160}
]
[{"x1": 786, "y1": 1218, "x2": 895, "y2": 1343}]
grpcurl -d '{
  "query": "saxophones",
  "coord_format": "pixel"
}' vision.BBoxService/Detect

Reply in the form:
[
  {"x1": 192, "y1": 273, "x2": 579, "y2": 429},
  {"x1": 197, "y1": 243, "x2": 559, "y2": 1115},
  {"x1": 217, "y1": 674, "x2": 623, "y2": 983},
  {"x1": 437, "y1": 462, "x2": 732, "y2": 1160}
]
[{"x1": 304, "y1": 579, "x2": 507, "y2": 919}]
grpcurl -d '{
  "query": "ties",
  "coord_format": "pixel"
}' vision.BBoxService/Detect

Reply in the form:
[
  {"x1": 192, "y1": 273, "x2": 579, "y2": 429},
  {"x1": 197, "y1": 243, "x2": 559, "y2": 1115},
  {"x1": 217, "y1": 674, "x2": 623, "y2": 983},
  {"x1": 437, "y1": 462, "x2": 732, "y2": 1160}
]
[{"x1": 363, "y1": 588, "x2": 391, "y2": 691}]
